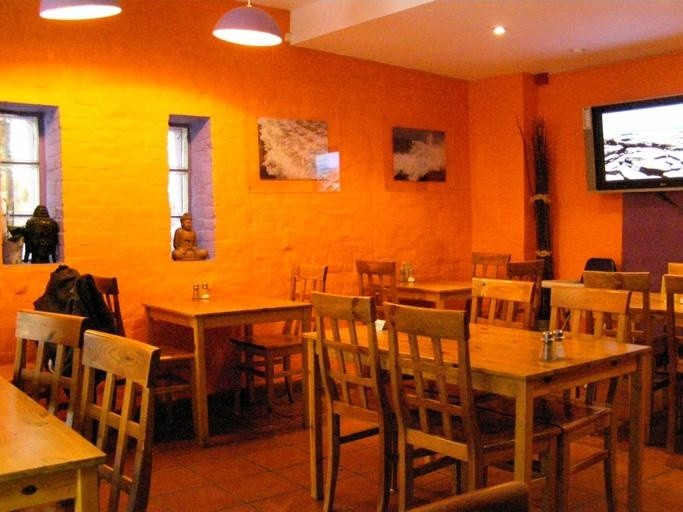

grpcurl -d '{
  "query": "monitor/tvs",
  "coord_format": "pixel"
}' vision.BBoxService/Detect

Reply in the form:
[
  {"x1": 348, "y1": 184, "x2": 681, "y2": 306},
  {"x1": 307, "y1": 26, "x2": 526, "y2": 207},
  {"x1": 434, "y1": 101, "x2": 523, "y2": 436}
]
[{"x1": 583, "y1": 94, "x2": 683, "y2": 194}]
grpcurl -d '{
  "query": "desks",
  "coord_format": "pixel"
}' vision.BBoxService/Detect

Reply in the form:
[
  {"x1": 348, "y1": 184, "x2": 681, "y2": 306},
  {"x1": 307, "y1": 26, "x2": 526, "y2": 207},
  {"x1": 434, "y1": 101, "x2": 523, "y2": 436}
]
[
  {"x1": 628, "y1": 291, "x2": 683, "y2": 320},
  {"x1": 301, "y1": 321, "x2": 654, "y2": 512},
  {"x1": 542, "y1": 280, "x2": 583, "y2": 289},
  {"x1": 373, "y1": 278, "x2": 472, "y2": 311},
  {"x1": 0, "y1": 374, "x2": 108, "y2": 512},
  {"x1": 141, "y1": 295, "x2": 314, "y2": 449}
]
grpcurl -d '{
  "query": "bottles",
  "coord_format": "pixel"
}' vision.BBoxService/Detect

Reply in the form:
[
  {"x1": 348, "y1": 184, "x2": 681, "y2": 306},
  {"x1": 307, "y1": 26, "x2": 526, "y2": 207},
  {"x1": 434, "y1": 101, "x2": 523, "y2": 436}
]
[
  {"x1": 539, "y1": 332, "x2": 552, "y2": 361},
  {"x1": 201, "y1": 283, "x2": 209, "y2": 298},
  {"x1": 552, "y1": 330, "x2": 564, "y2": 360},
  {"x1": 193, "y1": 284, "x2": 200, "y2": 298},
  {"x1": 398, "y1": 268, "x2": 405, "y2": 282},
  {"x1": 408, "y1": 268, "x2": 416, "y2": 282}
]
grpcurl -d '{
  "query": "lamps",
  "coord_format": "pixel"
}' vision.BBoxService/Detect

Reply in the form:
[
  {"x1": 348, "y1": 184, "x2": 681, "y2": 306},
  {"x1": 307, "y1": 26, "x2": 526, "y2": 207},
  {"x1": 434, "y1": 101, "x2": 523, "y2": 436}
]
[
  {"x1": 211, "y1": 0, "x2": 284, "y2": 47},
  {"x1": 38, "y1": 0, "x2": 124, "y2": 21}
]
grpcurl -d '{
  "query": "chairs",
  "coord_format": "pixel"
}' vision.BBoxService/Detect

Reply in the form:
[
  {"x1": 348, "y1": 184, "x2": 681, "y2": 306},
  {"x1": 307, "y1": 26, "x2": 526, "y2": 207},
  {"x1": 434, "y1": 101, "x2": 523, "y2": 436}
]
[
  {"x1": 506, "y1": 260, "x2": 546, "y2": 327},
  {"x1": 76, "y1": 328, "x2": 162, "y2": 512},
  {"x1": 230, "y1": 264, "x2": 328, "y2": 426},
  {"x1": 402, "y1": 277, "x2": 536, "y2": 400},
  {"x1": 311, "y1": 292, "x2": 463, "y2": 512},
  {"x1": 10, "y1": 309, "x2": 89, "y2": 428},
  {"x1": 660, "y1": 263, "x2": 683, "y2": 453},
  {"x1": 355, "y1": 260, "x2": 397, "y2": 307},
  {"x1": 93, "y1": 276, "x2": 200, "y2": 442},
  {"x1": 582, "y1": 270, "x2": 669, "y2": 448},
  {"x1": 476, "y1": 285, "x2": 632, "y2": 512},
  {"x1": 382, "y1": 301, "x2": 564, "y2": 512},
  {"x1": 581, "y1": 258, "x2": 617, "y2": 283},
  {"x1": 471, "y1": 253, "x2": 512, "y2": 320}
]
